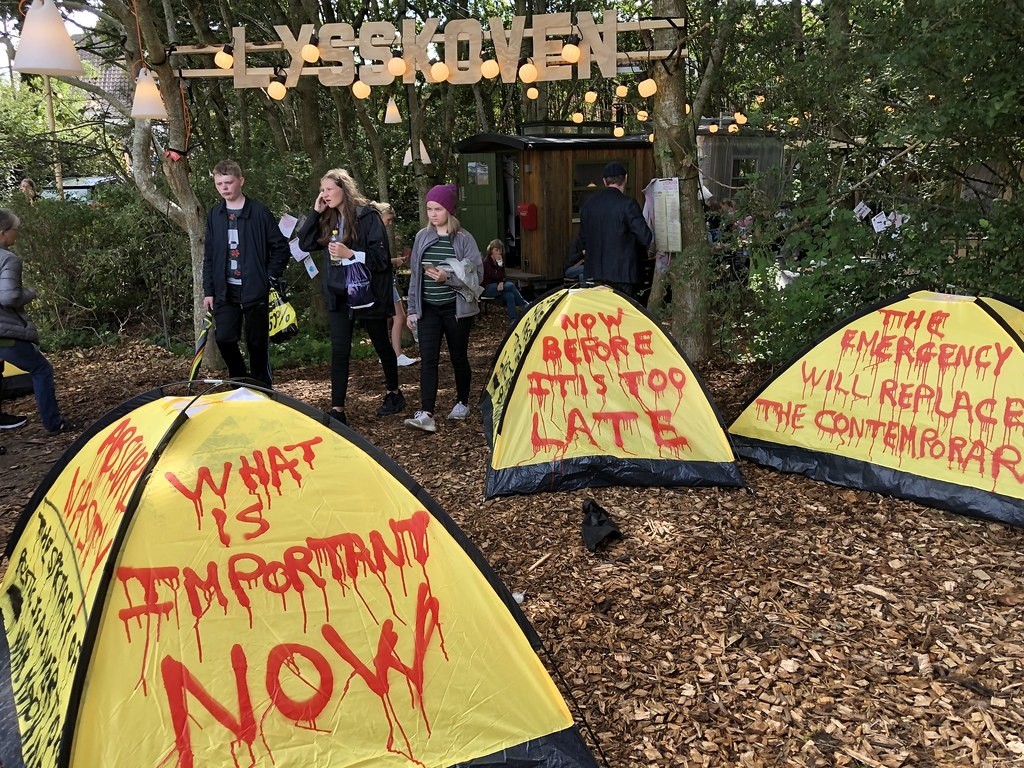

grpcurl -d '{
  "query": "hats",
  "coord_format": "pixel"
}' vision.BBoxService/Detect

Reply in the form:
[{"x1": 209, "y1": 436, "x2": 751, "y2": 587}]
[
  {"x1": 604, "y1": 162, "x2": 626, "y2": 178},
  {"x1": 425, "y1": 184, "x2": 456, "y2": 215}
]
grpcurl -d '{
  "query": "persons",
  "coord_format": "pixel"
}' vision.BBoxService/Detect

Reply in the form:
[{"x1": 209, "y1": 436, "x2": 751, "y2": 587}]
[
  {"x1": 645, "y1": 193, "x2": 802, "y2": 311},
  {"x1": 369, "y1": 200, "x2": 417, "y2": 367},
  {"x1": 406, "y1": 181, "x2": 485, "y2": 433},
  {"x1": 298, "y1": 168, "x2": 408, "y2": 426},
  {"x1": 479, "y1": 238, "x2": 533, "y2": 328},
  {"x1": 0, "y1": 357, "x2": 28, "y2": 429},
  {"x1": 202, "y1": 158, "x2": 290, "y2": 399},
  {"x1": 575, "y1": 161, "x2": 653, "y2": 309},
  {"x1": 0, "y1": 208, "x2": 82, "y2": 436},
  {"x1": 562, "y1": 230, "x2": 586, "y2": 289},
  {"x1": 19, "y1": 176, "x2": 45, "y2": 206}
]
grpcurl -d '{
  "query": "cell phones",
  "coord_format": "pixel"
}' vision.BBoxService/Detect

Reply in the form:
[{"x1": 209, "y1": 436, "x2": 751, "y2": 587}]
[{"x1": 421, "y1": 262, "x2": 435, "y2": 271}]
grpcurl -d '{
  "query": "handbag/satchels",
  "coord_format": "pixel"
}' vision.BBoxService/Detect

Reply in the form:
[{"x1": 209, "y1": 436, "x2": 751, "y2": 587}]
[
  {"x1": 345, "y1": 262, "x2": 375, "y2": 309},
  {"x1": 268, "y1": 280, "x2": 299, "y2": 346}
]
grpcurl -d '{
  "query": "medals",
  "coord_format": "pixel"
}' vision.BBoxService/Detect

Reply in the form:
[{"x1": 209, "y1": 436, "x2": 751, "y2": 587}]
[{"x1": 478, "y1": 283, "x2": 745, "y2": 503}]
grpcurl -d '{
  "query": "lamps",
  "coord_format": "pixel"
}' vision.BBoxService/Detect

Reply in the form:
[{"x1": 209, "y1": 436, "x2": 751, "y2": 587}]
[
  {"x1": 384, "y1": 91, "x2": 402, "y2": 124},
  {"x1": 403, "y1": 138, "x2": 431, "y2": 167},
  {"x1": 14, "y1": 0, "x2": 86, "y2": 76},
  {"x1": 130, "y1": 0, "x2": 167, "y2": 120}
]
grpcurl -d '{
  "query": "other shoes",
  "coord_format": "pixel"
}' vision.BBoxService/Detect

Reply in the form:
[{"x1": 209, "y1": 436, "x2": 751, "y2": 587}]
[
  {"x1": 510, "y1": 318, "x2": 516, "y2": 326},
  {"x1": 520, "y1": 300, "x2": 530, "y2": 311},
  {"x1": 0, "y1": 444, "x2": 7, "y2": 454}
]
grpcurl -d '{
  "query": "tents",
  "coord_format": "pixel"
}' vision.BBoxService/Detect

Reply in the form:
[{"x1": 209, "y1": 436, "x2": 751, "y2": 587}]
[
  {"x1": 725, "y1": 282, "x2": 1024, "y2": 535},
  {"x1": 0, "y1": 375, "x2": 615, "y2": 768}
]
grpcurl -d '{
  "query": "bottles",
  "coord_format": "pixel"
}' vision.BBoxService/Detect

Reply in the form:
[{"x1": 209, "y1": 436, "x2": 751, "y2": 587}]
[
  {"x1": 411, "y1": 317, "x2": 419, "y2": 342},
  {"x1": 329, "y1": 230, "x2": 341, "y2": 266}
]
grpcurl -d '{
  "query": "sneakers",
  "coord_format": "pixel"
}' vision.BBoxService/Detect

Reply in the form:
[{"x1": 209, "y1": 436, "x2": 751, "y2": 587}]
[
  {"x1": 448, "y1": 402, "x2": 470, "y2": 420},
  {"x1": 0, "y1": 414, "x2": 28, "y2": 428},
  {"x1": 405, "y1": 411, "x2": 436, "y2": 432},
  {"x1": 327, "y1": 409, "x2": 347, "y2": 425},
  {"x1": 379, "y1": 354, "x2": 416, "y2": 367},
  {"x1": 44, "y1": 415, "x2": 82, "y2": 437},
  {"x1": 376, "y1": 389, "x2": 407, "y2": 415}
]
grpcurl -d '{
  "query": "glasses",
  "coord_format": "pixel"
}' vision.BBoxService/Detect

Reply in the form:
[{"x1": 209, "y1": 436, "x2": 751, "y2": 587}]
[
  {"x1": 9, "y1": 226, "x2": 20, "y2": 233},
  {"x1": 381, "y1": 205, "x2": 393, "y2": 212}
]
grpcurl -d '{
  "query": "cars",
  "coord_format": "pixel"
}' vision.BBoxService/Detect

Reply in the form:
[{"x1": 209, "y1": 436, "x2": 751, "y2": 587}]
[{"x1": 36, "y1": 176, "x2": 116, "y2": 208}]
